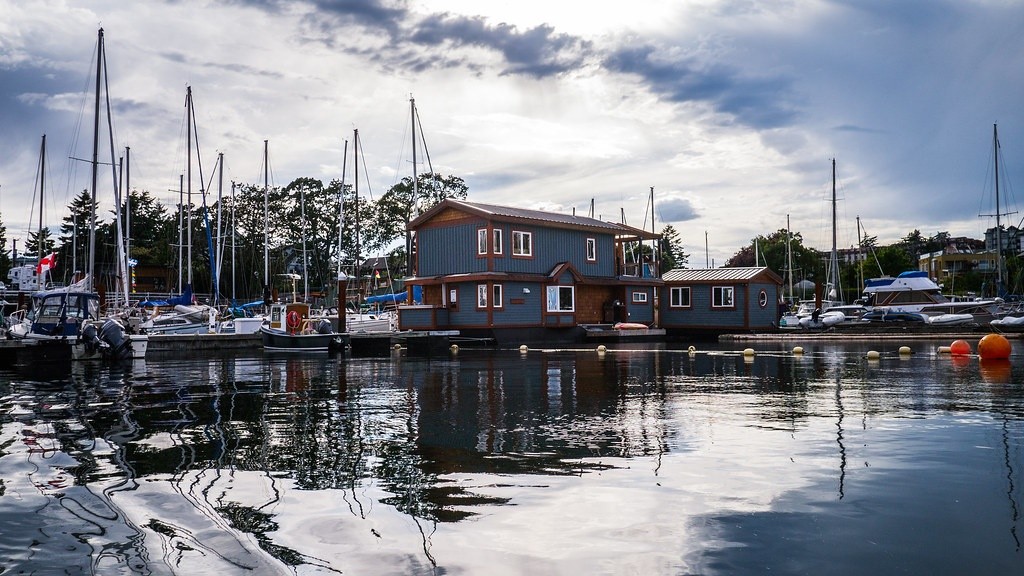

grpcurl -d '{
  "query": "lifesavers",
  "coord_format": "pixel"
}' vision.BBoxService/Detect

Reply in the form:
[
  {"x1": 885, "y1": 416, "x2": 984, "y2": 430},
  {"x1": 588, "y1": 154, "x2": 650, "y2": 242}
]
[{"x1": 287, "y1": 310, "x2": 300, "y2": 326}]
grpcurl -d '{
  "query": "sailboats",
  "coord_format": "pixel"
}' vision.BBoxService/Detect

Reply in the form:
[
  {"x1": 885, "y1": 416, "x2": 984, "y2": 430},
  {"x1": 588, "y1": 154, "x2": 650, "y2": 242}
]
[
  {"x1": 777, "y1": 124, "x2": 1023, "y2": 330},
  {"x1": 0, "y1": 28, "x2": 461, "y2": 372}
]
